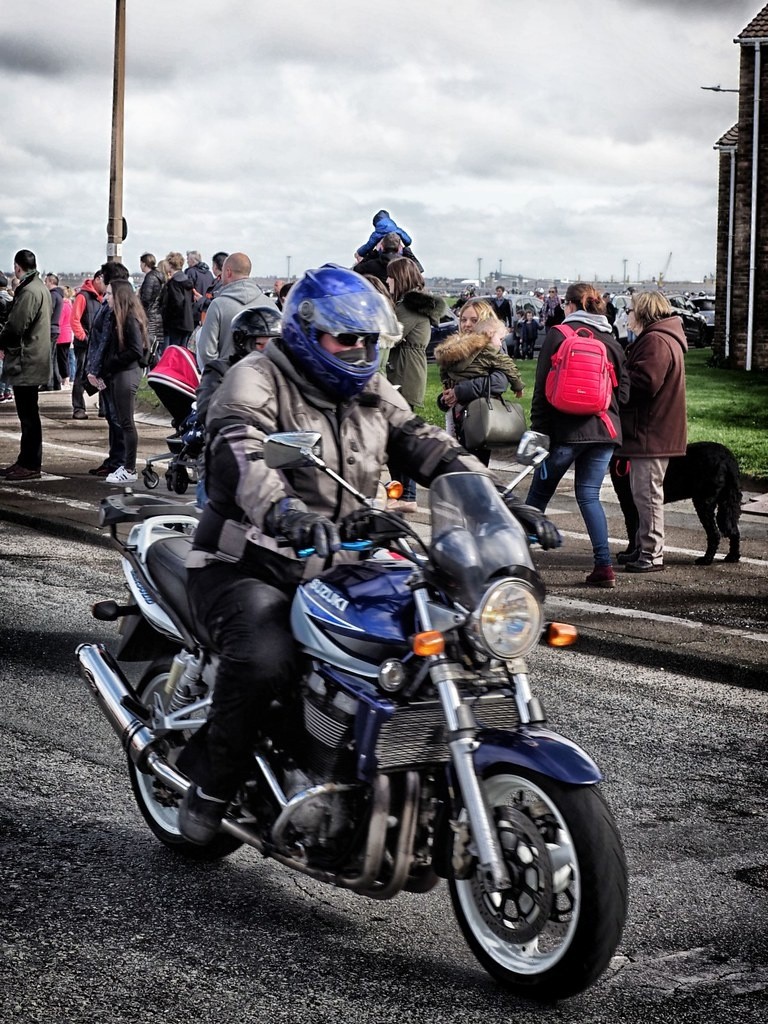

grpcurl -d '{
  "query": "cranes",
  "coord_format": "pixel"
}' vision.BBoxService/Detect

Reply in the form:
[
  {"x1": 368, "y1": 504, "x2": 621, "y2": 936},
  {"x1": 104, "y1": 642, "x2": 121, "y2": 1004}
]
[{"x1": 658, "y1": 250, "x2": 672, "y2": 292}]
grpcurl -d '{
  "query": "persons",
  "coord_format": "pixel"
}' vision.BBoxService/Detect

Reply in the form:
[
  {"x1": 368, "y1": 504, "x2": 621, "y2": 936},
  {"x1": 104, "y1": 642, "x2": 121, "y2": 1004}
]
[
  {"x1": 178, "y1": 263, "x2": 563, "y2": 848},
  {"x1": 0, "y1": 210, "x2": 709, "y2": 586}
]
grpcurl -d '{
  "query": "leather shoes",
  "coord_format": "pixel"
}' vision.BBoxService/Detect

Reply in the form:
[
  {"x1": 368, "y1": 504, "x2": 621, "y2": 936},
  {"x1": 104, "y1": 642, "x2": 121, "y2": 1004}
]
[
  {"x1": 617, "y1": 548, "x2": 641, "y2": 565},
  {"x1": 179, "y1": 782, "x2": 227, "y2": 846},
  {"x1": 626, "y1": 561, "x2": 666, "y2": 572}
]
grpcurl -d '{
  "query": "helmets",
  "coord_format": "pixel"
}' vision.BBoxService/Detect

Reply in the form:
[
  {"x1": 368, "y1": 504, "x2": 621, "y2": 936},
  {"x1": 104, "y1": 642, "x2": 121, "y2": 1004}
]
[
  {"x1": 230, "y1": 306, "x2": 281, "y2": 358},
  {"x1": 460, "y1": 290, "x2": 470, "y2": 301},
  {"x1": 465, "y1": 285, "x2": 476, "y2": 294},
  {"x1": 281, "y1": 263, "x2": 399, "y2": 401},
  {"x1": 534, "y1": 287, "x2": 545, "y2": 298}
]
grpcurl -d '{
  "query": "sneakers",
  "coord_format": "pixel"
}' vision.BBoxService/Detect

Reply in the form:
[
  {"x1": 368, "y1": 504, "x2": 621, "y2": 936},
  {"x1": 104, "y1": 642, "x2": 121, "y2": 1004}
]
[
  {"x1": 586, "y1": 563, "x2": 616, "y2": 587},
  {"x1": 106, "y1": 466, "x2": 139, "y2": 484}
]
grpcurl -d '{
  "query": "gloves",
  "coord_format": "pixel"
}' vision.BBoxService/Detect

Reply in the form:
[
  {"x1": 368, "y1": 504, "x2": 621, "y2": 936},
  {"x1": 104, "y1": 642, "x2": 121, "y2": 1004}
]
[
  {"x1": 465, "y1": 486, "x2": 563, "y2": 550},
  {"x1": 264, "y1": 498, "x2": 342, "y2": 558}
]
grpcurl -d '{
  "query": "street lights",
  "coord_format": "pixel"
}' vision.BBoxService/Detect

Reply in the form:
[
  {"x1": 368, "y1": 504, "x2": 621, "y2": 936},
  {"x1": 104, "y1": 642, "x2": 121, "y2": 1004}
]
[
  {"x1": 478, "y1": 258, "x2": 482, "y2": 288},
  {"x1": 287, "y1": 256, "x2": 291, "y2": 285},
  {"x1": 622, "y1": 259, "x2": 629, "y2": 291}
]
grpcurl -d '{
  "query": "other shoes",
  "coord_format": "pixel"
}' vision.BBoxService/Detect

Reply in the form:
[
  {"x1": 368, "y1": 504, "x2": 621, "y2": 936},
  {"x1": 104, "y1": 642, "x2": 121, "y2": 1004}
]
[
  {"x1": 0, "y1": 462, "x2": 41, "y2": 480},
  {"x1": 38, "y1": 382, "x2": 61, "y2": 391},
  {"x1": 89, "y1": 465, "x2": 117, "y2": 476},
  {"x1": 0, "y1": 392, "x2": 13, "y2": 403},
  {"x1": 72, "y1": 410, "x2": 88, "y2": 419}
]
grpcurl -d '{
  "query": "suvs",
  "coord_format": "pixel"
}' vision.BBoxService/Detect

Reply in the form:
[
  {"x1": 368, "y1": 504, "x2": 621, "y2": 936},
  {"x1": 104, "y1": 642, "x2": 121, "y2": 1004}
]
[
  {"x1": 690, "y1": 296, "x2": 715, "y2": 334},
  {"x1": 666, "y1": 295, "x2": 707, "y2": 349}
]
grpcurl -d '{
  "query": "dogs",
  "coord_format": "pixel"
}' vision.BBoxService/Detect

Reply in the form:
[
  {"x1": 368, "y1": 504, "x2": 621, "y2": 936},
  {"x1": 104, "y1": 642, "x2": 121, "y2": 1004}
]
[{"x1": 608, "y1": 438, "x2": 743, "y2": 567}]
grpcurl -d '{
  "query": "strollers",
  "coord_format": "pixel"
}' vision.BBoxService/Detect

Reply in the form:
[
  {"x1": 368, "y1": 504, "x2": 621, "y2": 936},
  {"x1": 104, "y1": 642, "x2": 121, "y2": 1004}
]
[{"x1": 142, "y1": 345, "x2": 202, "y2": 493}]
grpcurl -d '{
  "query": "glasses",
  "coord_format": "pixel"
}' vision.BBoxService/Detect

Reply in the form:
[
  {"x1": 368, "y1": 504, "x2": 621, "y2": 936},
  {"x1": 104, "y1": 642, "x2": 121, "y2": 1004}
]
[
  {"x1": 561, "y1": 302, "x2": 569, "y2": 310},
  {"x1": 337, "y1": 332, "x2": 373, "y2": 347},
  {"x1": 549, "y1": 291, "x2": 554, "y2": 293},
  {"x1": 625, "y1": 308, "x2": 634, "y2": 315}
]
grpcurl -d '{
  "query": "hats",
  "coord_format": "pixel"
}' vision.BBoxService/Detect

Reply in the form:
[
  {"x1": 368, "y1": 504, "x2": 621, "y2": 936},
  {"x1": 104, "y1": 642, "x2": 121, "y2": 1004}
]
[{"x1": 373, "y1": 210, "x2": 389, "y2": 226}]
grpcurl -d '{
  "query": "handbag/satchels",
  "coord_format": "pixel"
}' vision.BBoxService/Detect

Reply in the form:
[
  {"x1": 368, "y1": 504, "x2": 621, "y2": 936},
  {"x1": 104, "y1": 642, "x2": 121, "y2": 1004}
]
[{"x1": 463, "y1": 374, "x2": 526, "y2": 449}]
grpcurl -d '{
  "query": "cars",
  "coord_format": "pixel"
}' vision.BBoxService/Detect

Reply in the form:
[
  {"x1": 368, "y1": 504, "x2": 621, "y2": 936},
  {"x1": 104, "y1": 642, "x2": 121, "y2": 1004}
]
[
  {"x1": 471, "y1": 295, "x2": 547, "y2": 357},
  {"x1": 425, "y1": 313, "x2": 460, "y2": 358}
]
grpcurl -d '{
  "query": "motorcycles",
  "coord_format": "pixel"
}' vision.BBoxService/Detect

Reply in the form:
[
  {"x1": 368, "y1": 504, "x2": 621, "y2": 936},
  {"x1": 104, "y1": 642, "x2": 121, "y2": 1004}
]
[{"x1": 74, "y1": 432, "x2": 628, "y2": 1003}]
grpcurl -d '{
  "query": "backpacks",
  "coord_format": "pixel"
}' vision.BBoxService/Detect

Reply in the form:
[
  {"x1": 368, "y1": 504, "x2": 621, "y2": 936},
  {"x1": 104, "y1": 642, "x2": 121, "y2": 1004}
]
[{"x1": 546, "y1": 325, "x2": 617, "y2": 440}]
